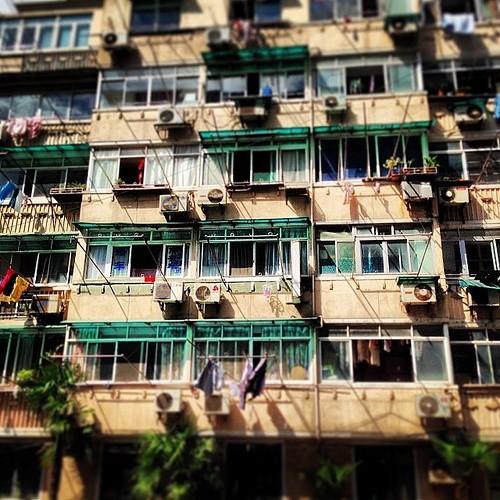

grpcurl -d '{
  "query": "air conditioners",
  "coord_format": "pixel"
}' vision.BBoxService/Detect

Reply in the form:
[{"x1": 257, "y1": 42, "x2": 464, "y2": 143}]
[
  {"x1": 193, "y1": 282, "x2": 221, "y2": 303},
  {"x1": 155, "y1": 390, "x2": 187, "y2": 413},
  {"x1": 100, "y1": 28, "x2": 130, "y2": 51},
  {"x1": 400, "y1": 281, "x2": 438, "y2": 307},
  {"x1": 400, "y1": 179, "x2": 434, "y2": 202},
  {"x1": 204, "y1": 392, "x2": 233, "y2": 416},
  {"x1": 30, "y1": 293, "x2": 62, "y2": 315},
  {"x1": 388, "y1": 17, "x2": 420, "y2": 35},
  {"x1": 454, "y1": 101, "x2": 488, "y2": 126},
  {"x1": 415, "y1": 393, "x2": 453, "y2": 418},
  {"x1": 323, "y1": 94, "x2": 347, "y2": 114},
  {"x1": 426, "y1": 460, "x2": 459, "y2": 488},
  {"x1": 154, "y1": 280, "x2": 185, "y2": 302},
  {"x1": 438, "y1": 187, "x2": 469, "y2": 207},
  {"x1": 157, "y1": 107, "x2": 186, "y2": 122},
  {"x1": 206, "y1": 27, "x2": 232, "y2": 45},
  {"x1": 237, "y1": 100, "x2": 267, "y2": 115},
  {"x1": 197, "y1": 185, "x2": 228, "y2": 207},
  {"x1": 160, "y1": 191, "x2": 190, "y2": 215}
]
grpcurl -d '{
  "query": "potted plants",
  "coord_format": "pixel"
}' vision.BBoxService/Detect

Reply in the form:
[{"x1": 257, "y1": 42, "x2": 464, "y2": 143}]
[
  {"x1": 405, "y1": 157, "x2": 416, "y2": 173},
  {"x1": 383, "y1": 156, "x2": 402, "y2": 178},
  {"x1": 423, "y1": 155, "x2": 441, "y2": 173}
]
